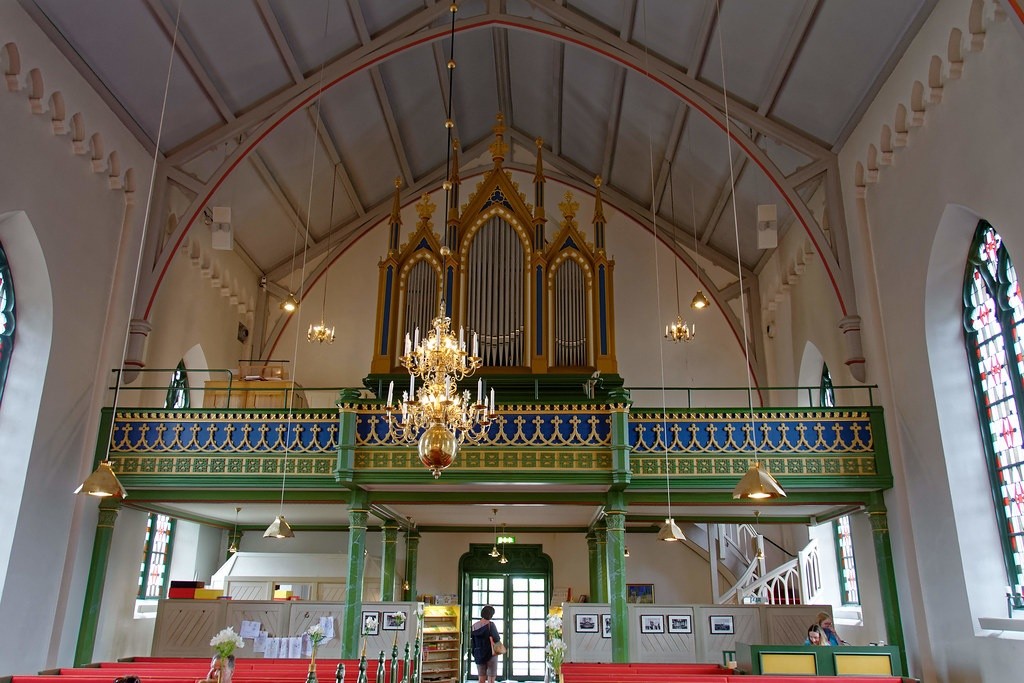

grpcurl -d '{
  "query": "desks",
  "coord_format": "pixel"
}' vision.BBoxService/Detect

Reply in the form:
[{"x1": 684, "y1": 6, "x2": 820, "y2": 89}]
[{"x1": 202, "y1": 381, "x2": 305, "y2": 408}]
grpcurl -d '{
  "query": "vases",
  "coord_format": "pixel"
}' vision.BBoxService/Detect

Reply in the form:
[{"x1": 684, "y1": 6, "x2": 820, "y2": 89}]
[
  {"x1": 400, "y1": 654, "x2": 409, "y2": 683},
  {"x1": 549, "y1": 672, "x2": 559, "y2": 683},
  {"x1": 356, "y1": 656, "x2": 368, "y2": 683},
  {"x1": 390, "y1": 646, "x2": 398, "y2": 683},
  {"x1": 410, "y1": 655, "x2": 419, "y2": 683},
  {"x1": 335, "y1": 663, "x2": 344, "y2": 683},
  {"x1": 376, "y1": 650, "x2": 385, "y2": 683},
  {"x1": 306, "y1": 672, "x2": 318, "y2": 683}
]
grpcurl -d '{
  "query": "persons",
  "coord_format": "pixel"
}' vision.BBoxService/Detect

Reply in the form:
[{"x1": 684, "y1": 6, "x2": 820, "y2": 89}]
[
  {"x1": 803, "y1": 610, "x2": 844, "y2": 646},
  {"x1": 471, "y1": 606, "x2": 501, "y2": 683}
]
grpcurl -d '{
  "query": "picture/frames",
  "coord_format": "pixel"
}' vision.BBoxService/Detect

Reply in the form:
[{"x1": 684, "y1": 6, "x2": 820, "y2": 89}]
[
  {"x1": 361, "y1": 611, "x2": 380, "y2": 636},
  {"x1": 626, "y1": 583, "x2": 655, "y2": 605},
  {"x1": 575, "y1": 614, "x2": 599, "y2": 633},
  {"x1": 602, "y1": 614, "x2": 612, "y2": 638},
  {"x1": 641, "y1": 615, "x2": 665, "y2": 634},
  {"x1": 667, "y1": 615, "x2": 692, "y2": 634},
  {"x1": 382, "y1": 612, "x2": 406, "y2": 631},
  {"x1": 709, "y1": 615, "x2": 734, "y2": 635}
]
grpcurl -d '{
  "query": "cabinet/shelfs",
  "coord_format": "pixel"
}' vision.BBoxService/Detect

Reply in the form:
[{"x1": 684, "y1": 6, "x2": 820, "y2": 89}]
[{"x1": 421, "y1": 604, "x2": 463, "y2": 683}]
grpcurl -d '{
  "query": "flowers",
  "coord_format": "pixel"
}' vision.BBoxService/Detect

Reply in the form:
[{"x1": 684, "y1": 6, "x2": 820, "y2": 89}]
[
  {"x1": 545, "y1": 613, "x2": 563, "y2": 640},
  {"x1": 544, "y1": 638, "x2": 567, "y2": 677},
  {"x1": 307, "y1": 623, "x2": 327, "y2": 647},
  {"x1": 413, "y1": 607, "x2": 425, "y2": 620},
  {"x1": 392, "y1": 610, "x2": 407, "y2": 626}
]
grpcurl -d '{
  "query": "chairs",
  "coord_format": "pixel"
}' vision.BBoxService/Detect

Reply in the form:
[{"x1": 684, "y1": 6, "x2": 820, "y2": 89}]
[{"x1": 238, "y1": 365, "x2": 283, "y2": 380}]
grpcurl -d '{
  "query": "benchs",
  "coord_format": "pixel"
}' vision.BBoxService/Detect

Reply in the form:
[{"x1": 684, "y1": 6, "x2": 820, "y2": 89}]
[
  {"x1": 558, "y1": 661, "x2": 921, "y2": 683},
  {"x1": 0, "y1": 657, "x2": 415, "y2": 683}
]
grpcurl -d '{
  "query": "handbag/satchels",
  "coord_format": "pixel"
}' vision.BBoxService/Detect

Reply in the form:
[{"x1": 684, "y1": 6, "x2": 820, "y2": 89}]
[{"x1": 493, "y1": 641, "x2": 507, "y2": 654}]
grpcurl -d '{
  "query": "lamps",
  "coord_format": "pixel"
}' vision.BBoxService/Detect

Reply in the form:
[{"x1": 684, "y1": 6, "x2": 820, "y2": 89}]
[
  {"x1": 382, "y1": 0, "x2": 500, "y2": 480},
  {"x1": 400, "y1": 516, "x2": 412, "y2": 592},
  {"x1": 488, "y1": 508, "x2": 509, "y2": 564},
  {"x1": 72, "y1": 0, "x2": 332, "y2": 555},
  {"x1": 624, "y1": 0, "x2": 788, "y2": 560}
]
